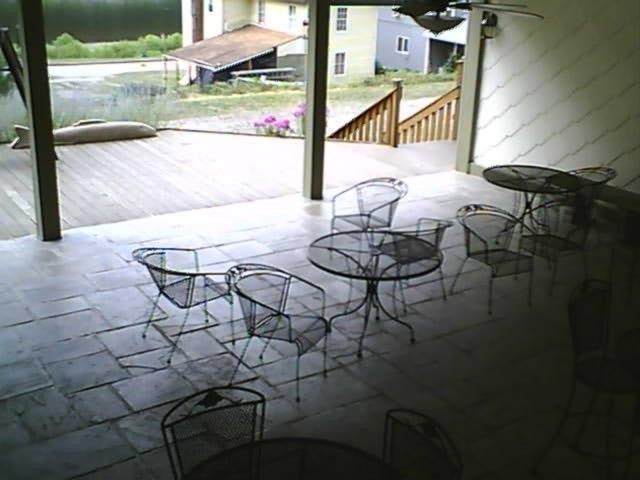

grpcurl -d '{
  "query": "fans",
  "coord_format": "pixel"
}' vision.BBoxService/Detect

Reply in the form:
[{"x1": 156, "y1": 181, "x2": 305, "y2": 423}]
[{"x1": 329, "y1": 0, "x2": 544, "y2": 19}]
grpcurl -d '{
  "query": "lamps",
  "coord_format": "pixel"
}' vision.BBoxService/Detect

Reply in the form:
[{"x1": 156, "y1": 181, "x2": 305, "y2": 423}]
[
  {"x1": 481, "y1": 13, "x2": 497, "y2": 38},
  {"x1": 415, "y1": 9, "x2": 464, "y2": 33}
]
